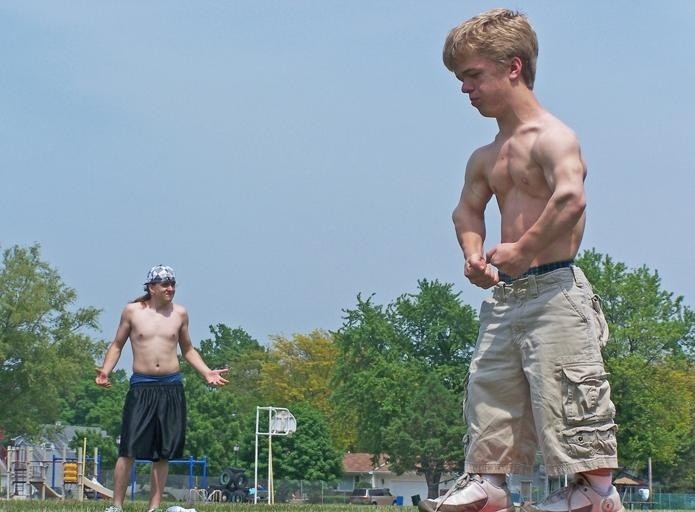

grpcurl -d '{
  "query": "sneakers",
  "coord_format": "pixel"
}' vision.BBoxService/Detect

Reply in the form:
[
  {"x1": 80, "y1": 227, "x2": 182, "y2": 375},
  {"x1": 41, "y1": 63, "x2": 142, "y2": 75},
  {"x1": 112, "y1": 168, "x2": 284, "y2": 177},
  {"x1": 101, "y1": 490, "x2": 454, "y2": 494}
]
[
  {"x1": 418, "y1": 474, "x2": 624, "y2": 512},
  {"x1": 105, "y1": 506, "x2": 123, "y2": 512},
  {"x1": 166, "y1": 506, "x2": 196, "y2": 512}
]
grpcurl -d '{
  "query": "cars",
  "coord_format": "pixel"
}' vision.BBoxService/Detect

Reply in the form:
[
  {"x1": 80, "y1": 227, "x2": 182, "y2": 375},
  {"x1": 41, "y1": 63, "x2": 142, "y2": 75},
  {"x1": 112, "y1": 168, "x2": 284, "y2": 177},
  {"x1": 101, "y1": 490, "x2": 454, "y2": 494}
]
[{"x1": 350, "y1": 486, "x2": 397, "y2": 506}]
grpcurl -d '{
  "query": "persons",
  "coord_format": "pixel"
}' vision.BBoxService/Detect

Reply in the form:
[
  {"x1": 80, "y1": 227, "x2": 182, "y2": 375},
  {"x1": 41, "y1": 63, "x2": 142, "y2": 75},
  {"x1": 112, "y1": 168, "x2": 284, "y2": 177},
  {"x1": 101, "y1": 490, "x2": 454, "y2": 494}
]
[
  {"x1": 414, "y1": 7, "x2": 626, "y2": 512},
  {"x1": 92, "y1": 263, "x2": 231, "y2": 512}
]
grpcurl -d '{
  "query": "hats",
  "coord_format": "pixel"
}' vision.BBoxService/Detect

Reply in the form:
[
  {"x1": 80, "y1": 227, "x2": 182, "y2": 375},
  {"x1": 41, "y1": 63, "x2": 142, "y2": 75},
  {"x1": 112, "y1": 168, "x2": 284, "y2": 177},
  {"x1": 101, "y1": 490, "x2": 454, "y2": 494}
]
[{"x1": 144, "y1": 265, "x2": 175, "y2": 284}]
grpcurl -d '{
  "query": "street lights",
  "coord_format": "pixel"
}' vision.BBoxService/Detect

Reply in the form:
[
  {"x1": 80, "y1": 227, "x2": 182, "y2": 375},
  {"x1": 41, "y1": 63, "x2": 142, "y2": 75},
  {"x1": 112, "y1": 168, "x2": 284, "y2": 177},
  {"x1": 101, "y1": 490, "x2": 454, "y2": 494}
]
[{"x1": 233, "y1": 443, "x2": 240, "y2": 466}]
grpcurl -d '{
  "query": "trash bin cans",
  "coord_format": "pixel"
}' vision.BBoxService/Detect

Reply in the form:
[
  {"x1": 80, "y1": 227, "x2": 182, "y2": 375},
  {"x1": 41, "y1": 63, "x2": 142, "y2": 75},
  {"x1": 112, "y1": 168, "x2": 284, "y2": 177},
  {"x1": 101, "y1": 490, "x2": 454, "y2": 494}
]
[
  {"x1": 411, "y1": 494, "x2": 421, "y2": 506},
  {"x1": 397, "y1": 496, "x2": 403, "y2": 506}
]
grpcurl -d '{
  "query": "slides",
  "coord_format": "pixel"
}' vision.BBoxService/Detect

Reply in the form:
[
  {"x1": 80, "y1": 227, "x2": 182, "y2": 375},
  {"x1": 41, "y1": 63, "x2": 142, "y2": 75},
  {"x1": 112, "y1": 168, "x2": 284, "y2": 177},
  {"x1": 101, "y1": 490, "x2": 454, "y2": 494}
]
[
  {"x1": 30, "y1": 483, "x2": 63, "y2": 499},
  {"x1": 81, "y1": 475, "x2": 114, "y2": 498}
]
